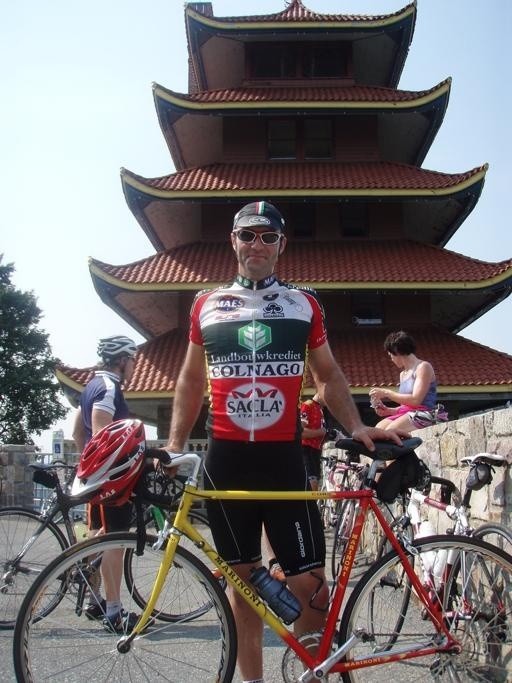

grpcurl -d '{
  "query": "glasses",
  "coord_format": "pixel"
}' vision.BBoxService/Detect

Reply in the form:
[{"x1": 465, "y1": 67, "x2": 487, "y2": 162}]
[{"x1": 235, "y1": 229, "x2": 283, "y2": 245}]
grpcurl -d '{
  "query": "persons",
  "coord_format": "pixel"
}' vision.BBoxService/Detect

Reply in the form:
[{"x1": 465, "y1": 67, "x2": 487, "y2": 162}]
[
  {"x1": 297, "y1": 390, "x2": 338, "y2": 507},
  {"x1": 150, "y1": 200, "x2": 413, "y2": 682},
  {"x1": 71, "y1": 333, "x2": 155, "y2": 633},
  {"x1": 369, "y1": 330, "x2": 449, "y2": 440}
]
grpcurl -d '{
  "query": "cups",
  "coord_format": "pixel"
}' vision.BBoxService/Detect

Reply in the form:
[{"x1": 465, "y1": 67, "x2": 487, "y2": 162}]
[{"x1": 73, "y1": 515, "x2": 89, "y2": 543}]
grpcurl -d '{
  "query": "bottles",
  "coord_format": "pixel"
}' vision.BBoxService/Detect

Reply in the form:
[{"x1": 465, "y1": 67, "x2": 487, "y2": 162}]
[
  {"x1": 249, "y1": 565, "x2": 304, "y2": 625},
  {"x1": 418, "y1": 518, "x2": 453, "y2": 578},
  {"x1": 151, "y1": 507, "x2": 165, "y2": 532}
]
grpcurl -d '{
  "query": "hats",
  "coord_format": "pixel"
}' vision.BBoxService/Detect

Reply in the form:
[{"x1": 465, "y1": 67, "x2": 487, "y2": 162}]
[{"x1": 234, "y1": 201, "x2": 285, "y2": 231}]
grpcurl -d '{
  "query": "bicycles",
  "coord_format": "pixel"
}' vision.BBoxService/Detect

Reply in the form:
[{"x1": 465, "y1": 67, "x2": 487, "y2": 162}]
[
  {"x1": 12, "y1": 435, "x2": 512, "y2": 683},
  {"x1": 320, "y1": 420, "x2": 512, "y2": 683},
  {"x1": 0, "y1": 449, "x2": 231, "y2": 630}
]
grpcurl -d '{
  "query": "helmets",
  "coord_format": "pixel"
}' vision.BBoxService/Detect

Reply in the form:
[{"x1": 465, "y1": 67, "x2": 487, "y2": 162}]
[
  {"x1": 72, "y1": 419, "x2": 146, "y2": 507},
  {"x1": 98, "y1": 335, "x2": 137, "y2": 359}
]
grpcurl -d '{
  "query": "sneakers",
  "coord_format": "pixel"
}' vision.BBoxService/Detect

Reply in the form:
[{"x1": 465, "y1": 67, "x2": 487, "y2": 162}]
[
  {"x1": 103, "y1": 609, "x2": 156, "y2": 634},
  {"x1": 270, "y1": 562, "x2": 286, "y2": 582},
  {"x1": 86, "y1": 598, "x2": 106, "y2": 619}
]
